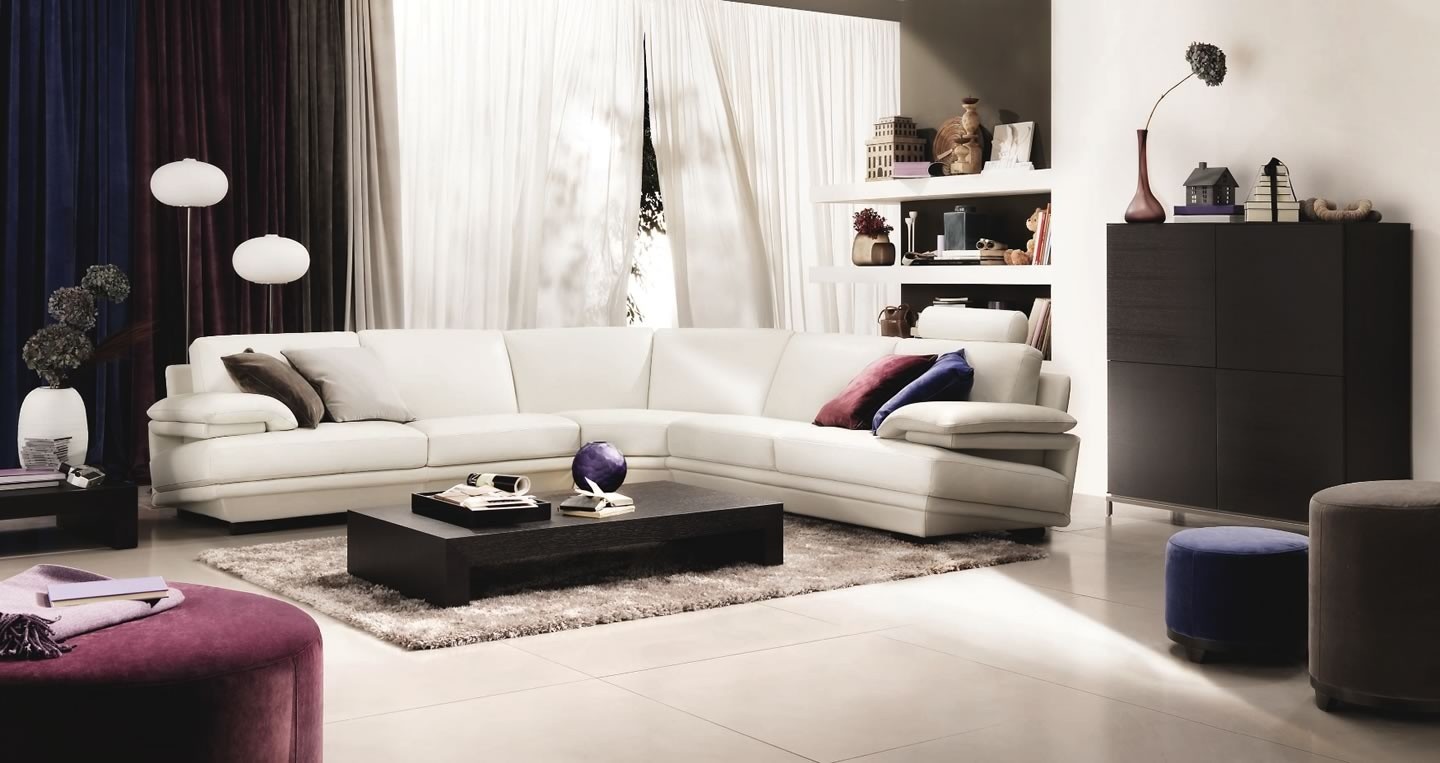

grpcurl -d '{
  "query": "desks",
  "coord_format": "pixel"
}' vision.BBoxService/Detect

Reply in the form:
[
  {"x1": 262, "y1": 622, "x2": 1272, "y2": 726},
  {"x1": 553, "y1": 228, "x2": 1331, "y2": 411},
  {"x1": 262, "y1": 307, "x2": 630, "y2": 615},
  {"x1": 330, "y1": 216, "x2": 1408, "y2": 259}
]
[{"x1": 0, "y1": 464, "x2": 138, "y2": 552}]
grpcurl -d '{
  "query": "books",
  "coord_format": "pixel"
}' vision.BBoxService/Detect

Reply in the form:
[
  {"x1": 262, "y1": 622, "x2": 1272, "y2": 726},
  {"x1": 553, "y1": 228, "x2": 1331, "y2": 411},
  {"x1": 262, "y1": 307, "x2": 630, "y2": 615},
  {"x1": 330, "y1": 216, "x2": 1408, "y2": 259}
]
[
  {"x1": 1026, "y1": 298, "x2": 1051, "y2": 360},
  {"x1": 1244, "y1": 164, "x2": 1300, "y2": 222},
  {"x1": 435, "y1": 472, "x2": 531, "y2": 506},
  {"x1": 48, "y1": 577, "x2": 170, "y2": 608},
  {"x1": 894, "y1": 161, "x2": 931, "y2": 177},
  {"x1": 1174, "y1": 205, "x2": 1244, "y2": 222},
  {"x1": 1032, "y1": 203, "x2": 1051, "y2": 265},
  {"x1": 912, "y1": 249, "x2": 1006, "y2": 265},
  {"x1": 559, "y1": 477, "x2": 635, "y2": 518},
  {"x1": 988, "y1": 301, "x2": 1015, "y2": 310},
  {"x1": 0, "y1": 436, "x2": 72, "y2": 484},
  {"x1": 933, "y1": 297, "x2": 976, "y2": 307}
]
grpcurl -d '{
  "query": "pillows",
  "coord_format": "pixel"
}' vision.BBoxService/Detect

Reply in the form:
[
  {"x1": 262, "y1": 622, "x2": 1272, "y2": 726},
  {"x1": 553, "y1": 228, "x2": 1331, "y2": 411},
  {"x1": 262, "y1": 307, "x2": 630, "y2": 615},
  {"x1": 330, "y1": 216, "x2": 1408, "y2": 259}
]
[
  {"x1": 873, "y1": 349, "x2": 975, "y2": 436},
  {"x1": 278, "y1": 344, "x2": 417, "y2": 423},
  {"x1": 221, "y1": 348, "x2": 326, "y2": 430},
  {"x1": 811, "y1": 354, "x2": 937, "y2": 431}
]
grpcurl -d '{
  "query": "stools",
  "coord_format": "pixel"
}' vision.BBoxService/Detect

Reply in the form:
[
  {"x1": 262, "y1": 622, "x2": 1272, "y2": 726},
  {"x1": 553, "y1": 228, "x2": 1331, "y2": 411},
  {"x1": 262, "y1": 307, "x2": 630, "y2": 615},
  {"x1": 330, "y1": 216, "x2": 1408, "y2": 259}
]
[
  {"x1": 1158, "y1": 525, "x2": 1310, "y2": 665},
  {"x1": 0, "y1": 584, "x2": 322, "y2": 763},
  {"x1": 1306, "y1": 477, "x2": 1439, "y2": 714}
]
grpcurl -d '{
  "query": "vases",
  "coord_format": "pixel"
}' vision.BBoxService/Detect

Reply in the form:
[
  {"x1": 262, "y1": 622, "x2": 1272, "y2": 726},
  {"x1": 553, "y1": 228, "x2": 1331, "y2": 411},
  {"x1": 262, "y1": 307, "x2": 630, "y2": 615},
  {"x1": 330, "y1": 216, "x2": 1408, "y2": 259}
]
[
  {"x1": 1125, "y1": 128, "x2": 1167, "y2": 223},
  {"x1": 851, "y1": 231, "x2": 890, "y2": 265}
]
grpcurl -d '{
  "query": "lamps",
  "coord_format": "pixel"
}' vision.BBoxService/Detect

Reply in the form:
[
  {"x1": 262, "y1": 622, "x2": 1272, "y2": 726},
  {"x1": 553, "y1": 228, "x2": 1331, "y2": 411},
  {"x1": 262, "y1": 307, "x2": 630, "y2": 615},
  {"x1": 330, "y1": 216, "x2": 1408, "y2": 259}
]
[
  {"x1": 151, "y1": 158, "x2": 229, "y2": 363},
  {"x1": 233, "y1": 233, "x2": 309, "y2": 333}
]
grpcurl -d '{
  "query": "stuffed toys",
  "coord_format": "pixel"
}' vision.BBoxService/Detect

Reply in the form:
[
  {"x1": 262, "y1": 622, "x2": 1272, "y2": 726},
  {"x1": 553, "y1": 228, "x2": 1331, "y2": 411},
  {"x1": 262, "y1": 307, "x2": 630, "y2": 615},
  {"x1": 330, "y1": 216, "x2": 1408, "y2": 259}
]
[{"x1": 1004, "y1": 208, "x2": 1046, "y2": 264}]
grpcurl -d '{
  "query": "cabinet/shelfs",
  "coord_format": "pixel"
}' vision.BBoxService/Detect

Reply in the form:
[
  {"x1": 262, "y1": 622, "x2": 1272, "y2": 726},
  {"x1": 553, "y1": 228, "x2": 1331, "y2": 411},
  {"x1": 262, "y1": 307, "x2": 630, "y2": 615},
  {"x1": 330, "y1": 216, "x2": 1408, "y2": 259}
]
[
  {"x1": 812, "y1": 167, "x2": 1050, "y2": 373},
  {"x1": 1102, "y1": 219, "x2": 1411, "y2": 529}
]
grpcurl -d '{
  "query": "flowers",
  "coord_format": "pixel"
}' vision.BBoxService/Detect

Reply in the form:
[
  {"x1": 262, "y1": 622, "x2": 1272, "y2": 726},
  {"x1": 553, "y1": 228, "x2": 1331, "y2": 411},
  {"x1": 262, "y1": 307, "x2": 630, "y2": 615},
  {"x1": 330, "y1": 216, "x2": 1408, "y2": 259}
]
[
  {"x1": 1145, "y1": 41, "x2": 1226, "y2": 130},
  {"x1": 852, "y1": 207, "x2": 892, "y2": 236}
]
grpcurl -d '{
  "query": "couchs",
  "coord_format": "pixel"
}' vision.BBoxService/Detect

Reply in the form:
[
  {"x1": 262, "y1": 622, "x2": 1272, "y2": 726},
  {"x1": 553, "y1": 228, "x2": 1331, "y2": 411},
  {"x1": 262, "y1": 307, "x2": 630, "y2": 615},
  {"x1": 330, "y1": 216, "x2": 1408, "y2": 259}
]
[{"x1": 151, "y1": 306, "x2": 1079, "y2": 538}]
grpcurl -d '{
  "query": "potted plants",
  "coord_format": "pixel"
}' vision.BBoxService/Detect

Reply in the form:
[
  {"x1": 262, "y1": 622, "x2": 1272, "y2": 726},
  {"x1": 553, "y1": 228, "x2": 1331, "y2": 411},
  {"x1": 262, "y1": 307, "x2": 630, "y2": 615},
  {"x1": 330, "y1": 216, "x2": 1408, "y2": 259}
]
[{"x1": 16, "y1": 263, "x2": 130, "y2": 468}]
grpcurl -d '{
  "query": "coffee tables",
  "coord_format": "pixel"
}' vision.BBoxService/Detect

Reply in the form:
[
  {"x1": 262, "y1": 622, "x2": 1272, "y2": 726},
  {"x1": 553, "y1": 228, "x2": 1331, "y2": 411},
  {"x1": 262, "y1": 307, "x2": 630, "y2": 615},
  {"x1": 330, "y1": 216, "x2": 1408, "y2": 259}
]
[{"x1": 346, "y1": 480, "x2": 783, "y2": 607}]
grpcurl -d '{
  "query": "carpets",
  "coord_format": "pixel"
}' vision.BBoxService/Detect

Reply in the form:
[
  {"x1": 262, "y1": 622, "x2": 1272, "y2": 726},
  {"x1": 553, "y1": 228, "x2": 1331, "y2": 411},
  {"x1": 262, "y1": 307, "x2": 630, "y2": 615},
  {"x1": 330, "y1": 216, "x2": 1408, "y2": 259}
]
[{"x1": 197, "y1": 508, "x2": 1049, "y2": 651}]
